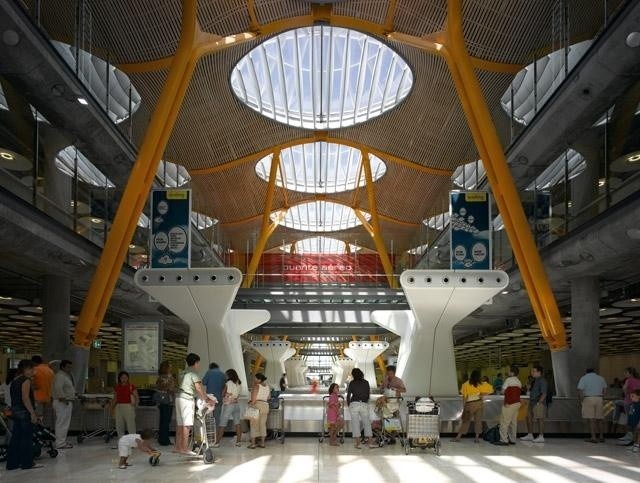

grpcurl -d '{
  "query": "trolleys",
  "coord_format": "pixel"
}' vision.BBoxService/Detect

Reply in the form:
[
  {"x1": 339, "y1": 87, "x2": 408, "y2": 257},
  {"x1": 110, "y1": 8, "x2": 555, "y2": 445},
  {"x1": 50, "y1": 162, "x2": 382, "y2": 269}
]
[
  {"x1": 366, "y1": 395, "x2": 446, "y2": 456},
  {"x1": 1, "y1": 389, "x2": 220, "y2": 471},
  {"x1": 316, "y1": 395, "x2": 347, "y2": 447},
  {"x1": 244, "y1": 395, "x2": 289, "y2": 444}
]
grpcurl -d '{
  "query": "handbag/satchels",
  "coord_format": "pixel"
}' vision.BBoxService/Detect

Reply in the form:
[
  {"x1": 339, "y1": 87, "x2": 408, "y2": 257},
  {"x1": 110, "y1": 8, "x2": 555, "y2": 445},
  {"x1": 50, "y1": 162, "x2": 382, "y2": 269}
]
[
  {"x1": 129, "y1": 383, "x2": 137, "y2": 407},
  {"x1": 482, "y1": 424, "x2": 508, "y2": 444},
  {"x1": 238, "y1": 405, "x2": 261, "y2": 422}
]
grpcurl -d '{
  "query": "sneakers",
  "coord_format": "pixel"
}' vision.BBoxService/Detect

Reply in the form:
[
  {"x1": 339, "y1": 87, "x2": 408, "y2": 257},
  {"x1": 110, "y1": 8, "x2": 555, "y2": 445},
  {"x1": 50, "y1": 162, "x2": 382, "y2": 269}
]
[
  {"x1": 531, "y1": 434, "x2": 545, "y2": 443},
  {"x1": 25, "y1": 442, "x2": 73, "y2": 469},
  {"x1": 328, "y1": 435, "x2": 397, "y2": 450},
  {"x1": 520, "y1": 433, "x2": 535, "y2": 442},
  {"x1": 472, "y1": 439, "x2": 480, "y2": 444},
  {"x1": 109, "y1": 441, "x2": 268, "y2": 449},
  {"x1": 493, "y1": 441, "x2": 510, "y2": 446},
  {"x1": 449, "y1": 438, "x2": 461, "y2": 442},
  {"x1": 118, "y1": 463, "x2": 127, "y2": 469},
  {"x1": 509, "y1": 439, "x2": 516, "y2": 445},
  {"x1": 585, "y1": 434, "x2": 640, "y2": 453},
  {"x1": 125, "y1": 461, "x2": 133, "y2": 467},
  {"x1": 179, "y1": 449, "x2": 198, "y2": 456}
]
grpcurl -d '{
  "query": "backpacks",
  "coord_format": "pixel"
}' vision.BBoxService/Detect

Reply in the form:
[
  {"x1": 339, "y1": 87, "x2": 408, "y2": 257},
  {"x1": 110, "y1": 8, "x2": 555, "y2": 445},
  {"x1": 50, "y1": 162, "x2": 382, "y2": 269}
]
[
  {"x1": 546, "y1": 380, "x2": 553, "y2": 405},
  {"x1": 152, "y1": 389, "x2": 171, "y2": 406}
]
